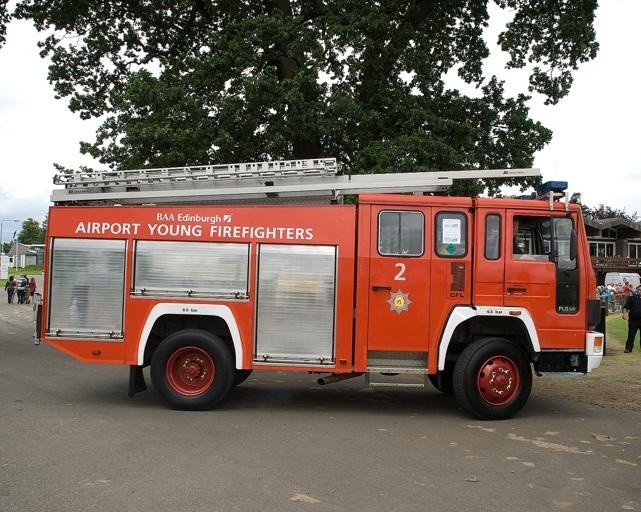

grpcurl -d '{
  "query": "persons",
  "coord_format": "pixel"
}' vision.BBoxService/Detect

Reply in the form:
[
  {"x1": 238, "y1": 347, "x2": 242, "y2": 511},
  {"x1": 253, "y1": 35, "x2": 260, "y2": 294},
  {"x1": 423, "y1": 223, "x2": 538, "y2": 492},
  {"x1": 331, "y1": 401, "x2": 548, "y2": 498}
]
[
  {"x1": 622, "y1": 284, "x2": 641, "y2": 353},
  {"x1": 594, "y1": 279, "x2": 638, "y2": 306},
  {"x1": 4, "y1": 273, "x2": 37, "y2": 304}
]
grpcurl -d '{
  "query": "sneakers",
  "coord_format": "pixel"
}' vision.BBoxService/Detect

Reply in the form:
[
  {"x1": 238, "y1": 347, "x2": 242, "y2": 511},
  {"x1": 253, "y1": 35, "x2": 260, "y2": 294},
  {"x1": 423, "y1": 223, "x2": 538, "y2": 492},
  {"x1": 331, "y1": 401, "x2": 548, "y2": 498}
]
[
  {"x1": 624, "y1": 349, "x2": 633, "y2": 353},
  {"x1": 8, "y1": 300, "x2": 30, "y2": 304}
]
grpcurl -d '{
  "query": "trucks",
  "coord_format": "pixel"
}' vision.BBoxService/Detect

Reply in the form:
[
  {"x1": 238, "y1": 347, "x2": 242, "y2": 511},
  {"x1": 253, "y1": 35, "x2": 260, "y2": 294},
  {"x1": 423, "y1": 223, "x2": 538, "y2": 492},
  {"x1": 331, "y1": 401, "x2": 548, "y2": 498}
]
[{"x1": 38, "y1": 153, "x2": 606, "y2": 422}]
